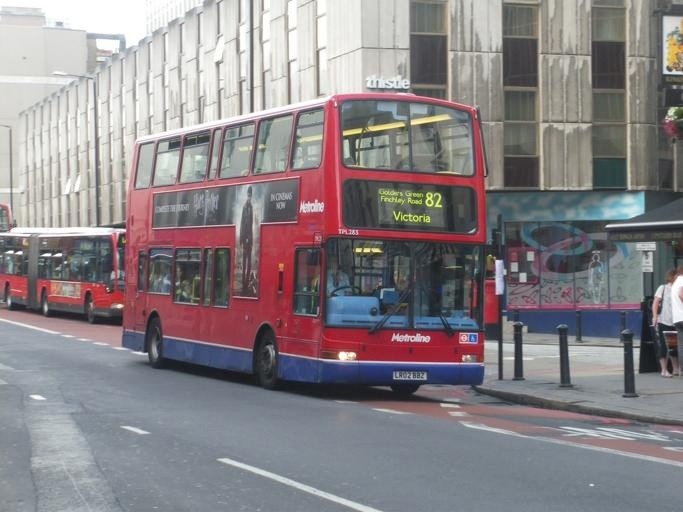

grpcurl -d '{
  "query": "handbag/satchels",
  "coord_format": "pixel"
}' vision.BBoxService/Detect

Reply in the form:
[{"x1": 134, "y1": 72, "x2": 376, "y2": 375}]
[{"x1": 646, "y1": 284, "x2": 666, "y2": 314}]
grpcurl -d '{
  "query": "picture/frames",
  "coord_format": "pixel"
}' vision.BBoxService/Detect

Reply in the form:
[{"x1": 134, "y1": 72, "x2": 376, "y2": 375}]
[{"x1": 658, "y1": 12, "x2": 683, "y2": 77}]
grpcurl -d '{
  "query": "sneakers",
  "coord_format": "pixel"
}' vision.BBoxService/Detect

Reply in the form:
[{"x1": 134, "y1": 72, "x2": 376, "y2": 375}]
[{"x1": 661, "y1": 372, "x2": 673, "y2": 378}]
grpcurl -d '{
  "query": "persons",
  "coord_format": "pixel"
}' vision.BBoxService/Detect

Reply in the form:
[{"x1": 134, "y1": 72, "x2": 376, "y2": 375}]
[
  {"x1": 670, "y1": 264, "x2": 682, "y2": 375},
  {"x1": 238, "y1": 185, "x2": 254, "y2": 290},
  {"x1": 179, "y1": 279, "x2": 191, "y2": 302},
  {"x1": 111, "y1": 261, "x2": 124, "y2": 280},
  {"x1": 317, "y1": 252, "x2": 352, "y2": 296},
  {"x1": 648, "y1": 268, "x2": 679, "y2": 377},
  {"x1": 173, "y1": 266, "x2": 183, "y2": 291}
]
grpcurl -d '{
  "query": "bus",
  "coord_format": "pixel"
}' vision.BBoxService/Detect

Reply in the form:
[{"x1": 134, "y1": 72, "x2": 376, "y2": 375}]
[
  {"x1": 116, "y1": 87, "x2": 495, "y2": 403},
  {"x1": 0, "y1": 223, "x2": 127, "y2": 323},
  {"x1": 0, "y1": 203, "x2": 17, "y2": 233}
]
